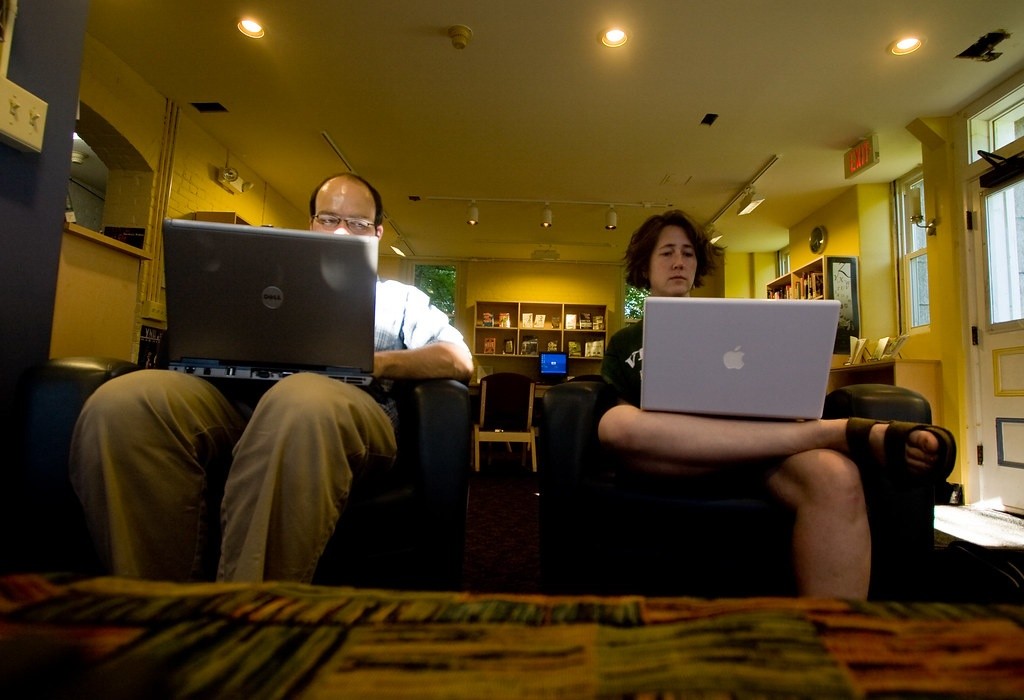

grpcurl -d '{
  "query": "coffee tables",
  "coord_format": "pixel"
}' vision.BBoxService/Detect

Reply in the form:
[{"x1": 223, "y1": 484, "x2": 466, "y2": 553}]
[{"x1": 0, "y1": 572, "x2": 1024, "y2": 700}]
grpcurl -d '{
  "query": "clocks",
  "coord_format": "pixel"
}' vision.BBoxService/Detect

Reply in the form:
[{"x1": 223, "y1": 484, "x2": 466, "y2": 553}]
[{"x1": 809, "y1": 225, "x2": 827, "y2": 254}]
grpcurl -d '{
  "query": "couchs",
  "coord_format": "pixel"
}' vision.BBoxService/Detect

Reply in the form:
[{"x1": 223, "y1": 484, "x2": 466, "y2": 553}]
[
  {"x1": 5, "y1": 356, "x2": 470, "y2": 593},
  {"x1": 537, "y1": 372, "x2": 934, "y2": 602}
]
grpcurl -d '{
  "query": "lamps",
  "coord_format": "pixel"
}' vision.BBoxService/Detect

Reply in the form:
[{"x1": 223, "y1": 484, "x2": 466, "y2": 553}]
[
  {"x1": 465, "y1": 200, "x2": 479, "y2": 226},
  {"x1": 540, "y1": 202, "x2": 552, "y2": 228},
  {"x1": 736, "y1": 185, "x2": 767, "y2": 216},
  {"x1": 908, "y1": 188, "x2": 935, "y2": 236},
  {"x1": 218, "y1": 166, "x2": 256, "y2": 196},
  {"x1": 706, "y1": 223, "x2": 723, "y2": 245},
  {"x1": 605, "y1": 205, "x2": 617, "y2": 230}
]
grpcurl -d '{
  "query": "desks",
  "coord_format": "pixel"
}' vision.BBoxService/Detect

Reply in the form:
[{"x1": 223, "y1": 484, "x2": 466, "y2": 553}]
[
  {"x1": 827, "y1": 358, "x2": 941, "y2": 426},
  {"x1": 466, "y1": 382, "x2": 554, "y2": 398}
]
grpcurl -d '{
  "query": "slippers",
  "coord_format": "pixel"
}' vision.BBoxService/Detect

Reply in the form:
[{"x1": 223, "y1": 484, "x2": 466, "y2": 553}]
[{"x1": 846, "y1": 417, "x2": 956, "y2": 485}]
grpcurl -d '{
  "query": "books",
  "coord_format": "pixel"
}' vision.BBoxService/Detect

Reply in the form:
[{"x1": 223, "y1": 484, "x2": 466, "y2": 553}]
[
  {"x1": 767, "y1": 270, "x2": 822, "y2": 299},
  {"x1": 482, "y1": 311, "x2": 605, "y2": 356},
  {"x1": 849, "y1": 335, "x2": 910, "y2": 365},
  {"x1": 993, "y1": 503, "x2": 1024, "y2": 515}
]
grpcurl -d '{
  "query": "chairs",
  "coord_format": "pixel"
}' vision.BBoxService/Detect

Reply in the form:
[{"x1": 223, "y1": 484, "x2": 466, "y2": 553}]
[{"x1": 474, "y1": 372, "x2": 538, "y2": 473}]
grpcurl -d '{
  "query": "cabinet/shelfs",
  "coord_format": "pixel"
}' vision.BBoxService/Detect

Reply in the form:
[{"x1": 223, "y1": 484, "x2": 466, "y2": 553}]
[
  {"x1": 767, "y1": 255, "x2": 861, "y2": 359},
  {"x1": 472, "y1": 299, "x2": 608, "y2": 360}
]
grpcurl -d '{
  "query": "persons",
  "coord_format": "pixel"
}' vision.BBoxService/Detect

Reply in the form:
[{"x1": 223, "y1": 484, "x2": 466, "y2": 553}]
[
  {"x1": 592, "y1": 210, "x2": 956, "y2": 601},
  {"x1": 68, "y1": 172, "x2": 474, "y2": 586}
]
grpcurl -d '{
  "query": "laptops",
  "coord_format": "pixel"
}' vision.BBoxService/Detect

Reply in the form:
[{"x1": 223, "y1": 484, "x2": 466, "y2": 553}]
[
  {"x1": 161, "y1": 218, "x2": 379, "y2": 386},
  {"x1": 639, "y1": 297, "x2": 841, "y2": 421}
]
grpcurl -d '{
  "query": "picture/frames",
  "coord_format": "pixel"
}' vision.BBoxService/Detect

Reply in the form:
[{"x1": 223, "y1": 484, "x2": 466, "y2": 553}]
[{"x1": 504, "y1": 337, "x2": 513, "y2": 354}]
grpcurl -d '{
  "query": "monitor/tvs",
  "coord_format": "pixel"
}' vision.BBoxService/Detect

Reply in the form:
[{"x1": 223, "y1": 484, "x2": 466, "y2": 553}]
[{"x1": 537, "y1": 351, "x2": 569, "y2": 385}]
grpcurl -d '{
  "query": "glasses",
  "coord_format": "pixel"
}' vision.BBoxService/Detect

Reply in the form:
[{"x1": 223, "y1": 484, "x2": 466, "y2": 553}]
[{"x1": 312, "y1": 215, "x2": 374, "y2": 235}]
